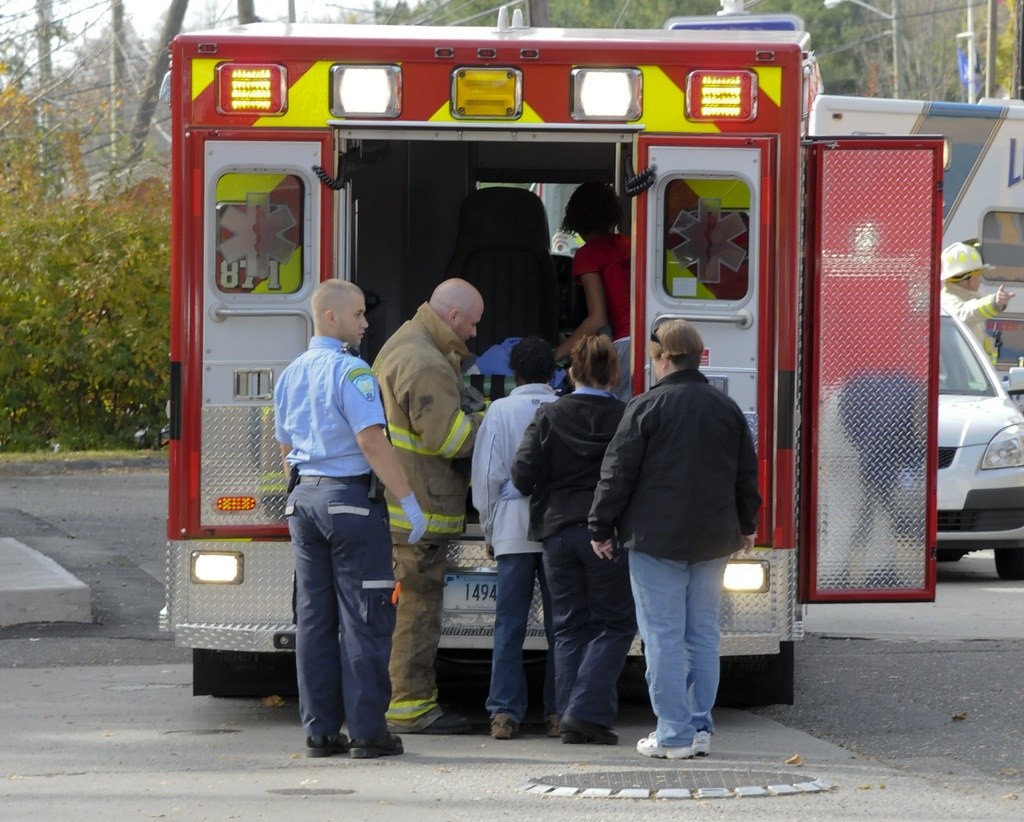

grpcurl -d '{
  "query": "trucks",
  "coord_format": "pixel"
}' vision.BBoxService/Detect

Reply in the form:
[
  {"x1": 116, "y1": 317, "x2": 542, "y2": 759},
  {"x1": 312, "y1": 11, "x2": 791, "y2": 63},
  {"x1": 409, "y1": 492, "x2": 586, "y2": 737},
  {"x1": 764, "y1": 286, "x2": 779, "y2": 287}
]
[{"x1": 804, "y1": 92, "x2": 1023, "y2": 405}]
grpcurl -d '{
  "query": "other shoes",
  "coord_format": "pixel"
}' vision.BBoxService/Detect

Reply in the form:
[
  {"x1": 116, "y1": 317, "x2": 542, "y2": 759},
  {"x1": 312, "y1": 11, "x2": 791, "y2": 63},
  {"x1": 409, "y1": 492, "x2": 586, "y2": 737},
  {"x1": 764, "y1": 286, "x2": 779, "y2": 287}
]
[
  {"x1": 558, "y1": 717, "x2": 619, "y2": 744},
  {"x1": 492, "y1": 714, "x2": 518, "y2": 738},
  {"x1": 544, "y1": 715, "x2": 559, "y2": 737}
]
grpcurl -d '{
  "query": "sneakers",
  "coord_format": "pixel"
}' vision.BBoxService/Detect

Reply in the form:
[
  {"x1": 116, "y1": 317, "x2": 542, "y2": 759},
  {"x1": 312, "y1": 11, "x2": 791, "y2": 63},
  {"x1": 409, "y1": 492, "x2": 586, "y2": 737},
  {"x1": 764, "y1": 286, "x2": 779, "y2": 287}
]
[
  {"x1": 636, "y1": 734, "x2": 694, "y2": 760},
  {"x1": 649, "y1": 730, "x2": 713, "y2": 757}
]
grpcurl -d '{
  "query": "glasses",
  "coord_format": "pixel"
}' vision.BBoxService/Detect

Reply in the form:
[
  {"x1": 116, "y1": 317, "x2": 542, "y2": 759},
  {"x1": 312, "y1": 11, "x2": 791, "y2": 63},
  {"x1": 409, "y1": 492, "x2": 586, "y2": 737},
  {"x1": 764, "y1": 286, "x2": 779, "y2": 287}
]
[{"x1": 650, "y1": 328, "x2": 662, "y2": 346}]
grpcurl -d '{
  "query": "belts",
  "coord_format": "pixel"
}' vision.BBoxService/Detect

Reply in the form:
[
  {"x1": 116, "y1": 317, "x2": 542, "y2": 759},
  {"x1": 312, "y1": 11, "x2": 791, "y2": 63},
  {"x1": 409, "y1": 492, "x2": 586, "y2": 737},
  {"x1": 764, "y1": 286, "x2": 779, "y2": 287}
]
[{"x1": 298, "y1": 475, "x2": 366, "y2": 483}]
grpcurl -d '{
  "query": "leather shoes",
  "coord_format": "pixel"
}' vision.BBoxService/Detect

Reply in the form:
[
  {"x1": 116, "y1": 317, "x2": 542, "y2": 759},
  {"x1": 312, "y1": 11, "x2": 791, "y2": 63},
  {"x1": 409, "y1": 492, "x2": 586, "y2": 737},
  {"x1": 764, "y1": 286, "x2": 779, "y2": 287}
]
[
  {"x1": 306, "y1": 731, "x2": 348, "y2": 757},
  {"x1": 417, "y1": 715, "x2": 473, "y2": 733},
  {"x1": 350, "y1": 729, "x2": 404, "y2": 757}
]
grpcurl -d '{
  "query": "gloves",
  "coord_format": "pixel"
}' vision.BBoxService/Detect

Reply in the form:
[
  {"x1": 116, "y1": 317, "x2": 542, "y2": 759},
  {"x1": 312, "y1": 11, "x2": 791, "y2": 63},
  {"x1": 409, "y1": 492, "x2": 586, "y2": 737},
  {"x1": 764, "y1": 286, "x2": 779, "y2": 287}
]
[{"x1": 398, "y1": 491, "x2": 427, "y2": 545}]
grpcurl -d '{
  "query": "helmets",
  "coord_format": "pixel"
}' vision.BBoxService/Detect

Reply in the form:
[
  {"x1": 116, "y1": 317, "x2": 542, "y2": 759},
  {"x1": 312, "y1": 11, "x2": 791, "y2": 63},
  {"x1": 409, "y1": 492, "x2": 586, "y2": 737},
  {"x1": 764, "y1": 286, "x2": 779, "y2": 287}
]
[{"x1": 939, "y1": 242, "x2": 988, "y2": 282}]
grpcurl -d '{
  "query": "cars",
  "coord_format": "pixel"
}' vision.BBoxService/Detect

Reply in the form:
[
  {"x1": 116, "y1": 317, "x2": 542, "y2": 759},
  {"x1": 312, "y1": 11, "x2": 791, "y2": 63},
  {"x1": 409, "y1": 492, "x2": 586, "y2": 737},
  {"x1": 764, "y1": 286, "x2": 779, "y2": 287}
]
[{"x1": 938, "y1": 306, "x2": 1024, "y2": 579}]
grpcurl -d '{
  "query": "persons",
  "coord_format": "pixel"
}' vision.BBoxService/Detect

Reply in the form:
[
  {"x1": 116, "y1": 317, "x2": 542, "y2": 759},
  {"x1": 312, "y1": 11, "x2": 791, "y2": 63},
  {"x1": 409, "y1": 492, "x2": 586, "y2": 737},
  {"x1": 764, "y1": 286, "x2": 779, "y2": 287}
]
[
  {"x1": 558, "y1": 177, "x2": 631, "y2": 405},
  {"x1": 588, "y1": 316, "x2": 764, "y2": 760},
  {"x1": 371, "y1": 278, "x2": 491, "y2": 734},
  {"x1": 940, "y1": 241, "x2": 1016, "y2": 365},
  {"x1": 471, "y1": 333, "x2": 562, "y2": 739},
  {"x1": 510, "y1": 334, "x2": 638, "y2": 744},
  {"x1": 274, "y1": 277, "x2": 426, "y2": 758}
]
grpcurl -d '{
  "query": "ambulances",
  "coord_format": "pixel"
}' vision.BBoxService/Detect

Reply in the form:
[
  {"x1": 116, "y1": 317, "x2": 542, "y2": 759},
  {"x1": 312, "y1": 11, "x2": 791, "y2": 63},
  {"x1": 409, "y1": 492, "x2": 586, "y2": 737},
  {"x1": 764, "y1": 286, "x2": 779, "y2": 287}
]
[{"x1": 159, "y1": 0, "x2": 947, "y2": 710}]
